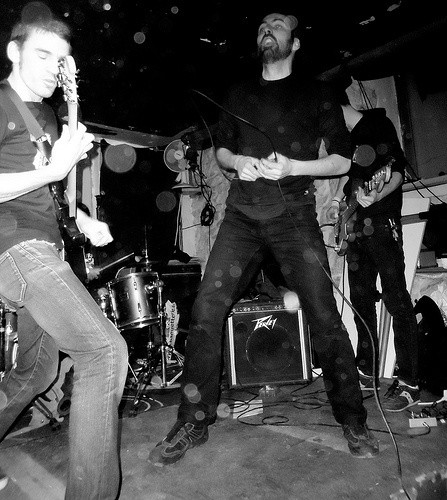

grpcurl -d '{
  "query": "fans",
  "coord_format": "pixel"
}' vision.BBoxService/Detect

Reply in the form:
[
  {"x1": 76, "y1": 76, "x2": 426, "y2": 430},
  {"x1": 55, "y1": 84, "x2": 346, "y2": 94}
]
[{"x1": 164, "y1": 140, "x2": 200, "y2": 188}]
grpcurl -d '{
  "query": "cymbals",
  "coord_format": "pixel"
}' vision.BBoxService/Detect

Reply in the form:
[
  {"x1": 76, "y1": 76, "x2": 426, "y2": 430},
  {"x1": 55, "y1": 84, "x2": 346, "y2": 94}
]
[
  {"x1": 126, "y1": 260, "x2": 168, "y2": 264},
  {"x1": 90, "y1": 289, "x2": 124, "y2": 333}
]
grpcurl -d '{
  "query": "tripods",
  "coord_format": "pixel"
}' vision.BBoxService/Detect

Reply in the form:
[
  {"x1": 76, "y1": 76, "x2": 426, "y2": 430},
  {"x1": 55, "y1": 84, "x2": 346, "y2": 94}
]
[{"x1": 129, "y1": 287, "x2": 185, "y2": 418}]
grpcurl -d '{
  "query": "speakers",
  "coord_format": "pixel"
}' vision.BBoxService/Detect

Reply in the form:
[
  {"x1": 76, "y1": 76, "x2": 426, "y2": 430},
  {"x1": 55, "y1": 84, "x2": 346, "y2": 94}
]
[{"x1": 224, "y1": 300, "x2": 313, "y2": 389}]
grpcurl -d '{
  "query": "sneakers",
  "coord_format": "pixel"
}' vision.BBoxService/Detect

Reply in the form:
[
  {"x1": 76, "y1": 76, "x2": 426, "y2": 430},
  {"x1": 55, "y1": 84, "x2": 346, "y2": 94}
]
[
  {"x1": 355, "y1": 366, "x2": 381, "y2": 392},
  {"x1": 375, "y1": 380, "x2": 421, "y2": 413},
  {"x1": 338, "y1": 412, "x2": 381, "y2": 460},
  {"x1": 146, "y1": 419, "x2": 211, "y2": 468}
]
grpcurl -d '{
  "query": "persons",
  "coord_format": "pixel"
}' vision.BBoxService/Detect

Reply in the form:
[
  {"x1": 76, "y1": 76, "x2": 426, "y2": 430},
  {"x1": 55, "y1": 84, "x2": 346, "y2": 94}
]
[
  {"x1": 0, "y1": 15, "x2": 128, "y2": 500},
  {"x1": 326, "y1": 87, "x2": 421, "y2": 412},
  {"x1": 75, "y1": 236, "x2": 114, "y2": 281},
  {"x1": 148, "y1": 10, "x2": 380, "y2": 464}
]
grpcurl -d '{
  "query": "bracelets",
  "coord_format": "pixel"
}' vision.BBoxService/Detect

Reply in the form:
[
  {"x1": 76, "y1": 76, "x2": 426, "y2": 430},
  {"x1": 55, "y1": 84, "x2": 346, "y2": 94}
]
[{"x1": 332, "y1": 199, "x2": 340, "y2": 204}]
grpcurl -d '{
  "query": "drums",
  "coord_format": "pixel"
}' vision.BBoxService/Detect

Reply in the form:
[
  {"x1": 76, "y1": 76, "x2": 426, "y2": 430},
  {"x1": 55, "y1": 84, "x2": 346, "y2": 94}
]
[{"x1": 110, "y1": 272, "x2": 164, "y2": 328}]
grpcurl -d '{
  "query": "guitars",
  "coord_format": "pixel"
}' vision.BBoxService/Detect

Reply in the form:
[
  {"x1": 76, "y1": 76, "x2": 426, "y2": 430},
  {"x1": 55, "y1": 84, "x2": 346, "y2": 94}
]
[
  {"x1": 334, "y1": 163, "x2": 390, "y2": 257},
  {"x1": 54, "y1": 54, "x2": 88, "y2": 289}
]
